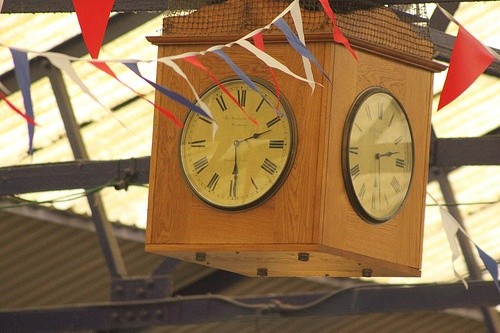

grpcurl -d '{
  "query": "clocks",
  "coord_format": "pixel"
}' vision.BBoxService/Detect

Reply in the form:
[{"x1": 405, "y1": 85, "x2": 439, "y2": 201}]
[
  {"x1": 178, "y1": 73, "x2": 299, "y2": 213},
  {"x1": 341, "y1": 84, "x2": 415, "y2": 225}
]
